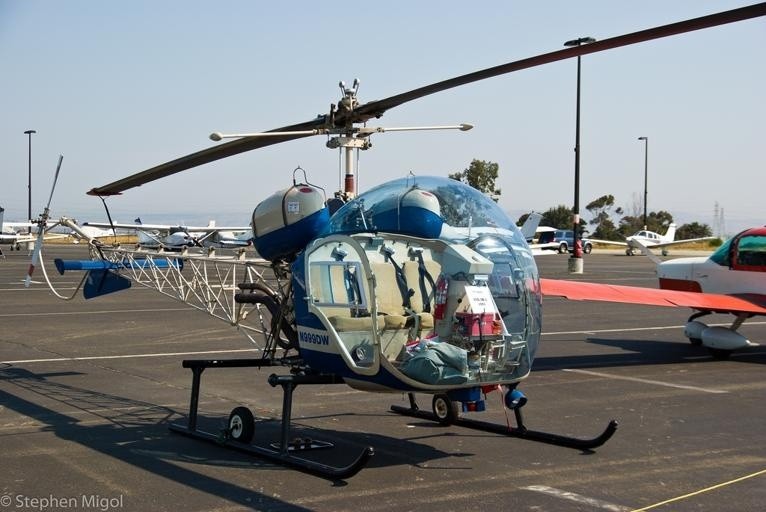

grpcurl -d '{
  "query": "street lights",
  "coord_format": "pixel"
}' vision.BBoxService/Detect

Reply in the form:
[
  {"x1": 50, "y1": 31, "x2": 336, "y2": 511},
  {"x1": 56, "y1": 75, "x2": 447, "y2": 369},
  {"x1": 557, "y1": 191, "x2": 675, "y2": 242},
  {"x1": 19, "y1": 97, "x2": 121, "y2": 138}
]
[
  {"x1": 23, "y1": 130, "x2": 36, "y2": 236},
  {"x1": 638, "y1": 136, "x2": 648, "y2": 230},
  {"x1": 564, "y1": 36, "x2": 597, "y2": 258}
]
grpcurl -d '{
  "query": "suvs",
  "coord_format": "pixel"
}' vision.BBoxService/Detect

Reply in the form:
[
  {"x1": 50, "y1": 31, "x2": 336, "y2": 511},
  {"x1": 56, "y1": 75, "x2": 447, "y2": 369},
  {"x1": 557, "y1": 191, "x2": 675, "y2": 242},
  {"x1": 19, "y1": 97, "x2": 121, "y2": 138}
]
[{"x1": 538, "y1": 230, "x2": 593, "y2": 254}]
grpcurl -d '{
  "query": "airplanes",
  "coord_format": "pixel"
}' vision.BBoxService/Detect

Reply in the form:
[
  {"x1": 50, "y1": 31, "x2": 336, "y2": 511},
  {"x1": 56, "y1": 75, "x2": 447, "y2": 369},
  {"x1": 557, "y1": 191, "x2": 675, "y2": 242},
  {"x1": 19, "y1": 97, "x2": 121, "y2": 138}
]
[
  {"x1": 580, "y1": 222, "x2": 718, "y2": 256},
  {"x1": 630, "y1": 227, "x2": 766, "y2": 351},
  {"x1": 0, "y1": 207, "x2": 254, "y2": 256}
]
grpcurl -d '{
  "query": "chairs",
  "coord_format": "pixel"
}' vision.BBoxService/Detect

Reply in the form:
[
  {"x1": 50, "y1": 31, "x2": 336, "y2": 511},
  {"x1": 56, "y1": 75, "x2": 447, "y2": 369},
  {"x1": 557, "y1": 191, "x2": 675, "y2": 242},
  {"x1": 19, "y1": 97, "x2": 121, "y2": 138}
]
[{"x1": 308, "y1": 260, "x2": 442, "y2": 331}]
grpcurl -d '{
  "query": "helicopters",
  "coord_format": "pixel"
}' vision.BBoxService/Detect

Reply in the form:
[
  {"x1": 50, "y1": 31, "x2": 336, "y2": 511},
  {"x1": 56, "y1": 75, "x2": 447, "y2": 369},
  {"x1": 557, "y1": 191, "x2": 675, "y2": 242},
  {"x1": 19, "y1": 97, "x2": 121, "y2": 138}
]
[{"x1": 85, "y1": 1, "x2": 766, "y2": 481}]
